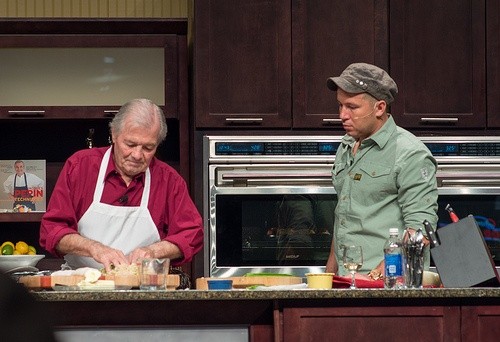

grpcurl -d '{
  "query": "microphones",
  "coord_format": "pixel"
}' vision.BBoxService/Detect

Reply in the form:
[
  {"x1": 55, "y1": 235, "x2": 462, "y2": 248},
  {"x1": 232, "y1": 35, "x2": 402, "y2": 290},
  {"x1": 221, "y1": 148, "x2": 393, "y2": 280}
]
[
  {"x1": 120, "y1": 195, "x2": 128, "y2": 202},
  {"x1": 354, "y1": 102, "x2": 377, "y2": 120}
]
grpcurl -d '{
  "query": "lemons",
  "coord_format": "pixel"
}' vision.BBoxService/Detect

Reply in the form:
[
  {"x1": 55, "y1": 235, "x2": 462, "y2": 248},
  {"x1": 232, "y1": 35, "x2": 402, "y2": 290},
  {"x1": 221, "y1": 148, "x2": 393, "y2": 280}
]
[{"x1": 0, "y1": 241, "x2": 37, "y2": 255}]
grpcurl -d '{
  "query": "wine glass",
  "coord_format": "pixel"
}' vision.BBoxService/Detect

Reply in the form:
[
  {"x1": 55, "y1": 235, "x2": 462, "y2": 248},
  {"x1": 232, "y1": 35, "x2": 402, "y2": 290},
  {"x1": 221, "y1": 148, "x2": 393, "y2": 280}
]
[{"x1": 343, "y1": 245, "x2": 363, "y2": 290}]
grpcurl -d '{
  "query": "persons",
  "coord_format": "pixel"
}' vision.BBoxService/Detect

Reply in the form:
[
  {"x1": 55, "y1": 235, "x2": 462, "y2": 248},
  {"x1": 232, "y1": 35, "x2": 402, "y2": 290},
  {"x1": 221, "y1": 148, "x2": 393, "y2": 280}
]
[
  {"x1": 39, "y1": 98, "x2": 204, "y2": 274},
  {"x1": 3, "y1": 161, "x2": 45, "y2": 211},
  {"x1": 327, "y1": 62, "x2": 439, "y2": 283}
]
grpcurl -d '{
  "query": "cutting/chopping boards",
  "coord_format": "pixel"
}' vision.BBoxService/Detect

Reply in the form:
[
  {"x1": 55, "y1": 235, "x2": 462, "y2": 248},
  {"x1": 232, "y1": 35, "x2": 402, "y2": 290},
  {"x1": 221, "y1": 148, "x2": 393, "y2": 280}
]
[
  {"x1": 197, "y1": 277, "x2": 303, "y2": 289},
  {"x1": 17, "y1": 274, "x2": 180, "y2": 291}
]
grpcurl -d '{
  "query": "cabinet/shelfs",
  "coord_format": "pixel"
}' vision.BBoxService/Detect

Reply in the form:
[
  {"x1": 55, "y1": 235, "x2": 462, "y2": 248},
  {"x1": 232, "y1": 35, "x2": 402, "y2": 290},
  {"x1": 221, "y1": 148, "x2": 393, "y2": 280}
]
[{"x1": 192, "y1": 0, "x2": 500, "y2": 136}]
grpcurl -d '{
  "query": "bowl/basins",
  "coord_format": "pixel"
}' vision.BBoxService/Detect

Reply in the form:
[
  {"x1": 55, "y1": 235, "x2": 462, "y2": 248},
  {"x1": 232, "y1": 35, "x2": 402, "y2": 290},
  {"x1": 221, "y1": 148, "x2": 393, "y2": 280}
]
[
  {"x1": 207, "y1": 279, "x2": 233, "y2": 290},
  {"x1": 305, "y1": 272, "x2": 335, "y2": 289}
]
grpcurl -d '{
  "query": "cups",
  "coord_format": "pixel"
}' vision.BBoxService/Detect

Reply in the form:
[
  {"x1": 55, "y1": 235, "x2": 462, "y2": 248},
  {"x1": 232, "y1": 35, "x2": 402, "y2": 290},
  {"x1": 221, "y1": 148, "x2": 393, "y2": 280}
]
[{"x1": 139, "y1": 258, "x2": 169, "y2": 290}]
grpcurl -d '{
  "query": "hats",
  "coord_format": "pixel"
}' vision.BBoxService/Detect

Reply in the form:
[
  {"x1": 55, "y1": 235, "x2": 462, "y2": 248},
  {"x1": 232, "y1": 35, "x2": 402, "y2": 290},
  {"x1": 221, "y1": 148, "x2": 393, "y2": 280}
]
[{"x1": 326, "y1": 63, "x2": 399, "y2": 104}]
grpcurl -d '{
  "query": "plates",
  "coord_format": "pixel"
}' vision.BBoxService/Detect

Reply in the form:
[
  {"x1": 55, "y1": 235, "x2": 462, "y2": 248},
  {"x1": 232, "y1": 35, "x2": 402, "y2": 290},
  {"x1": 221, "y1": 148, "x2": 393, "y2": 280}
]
[{"x1": 0, "y1": 255, "x2": 46, "y2": 274}]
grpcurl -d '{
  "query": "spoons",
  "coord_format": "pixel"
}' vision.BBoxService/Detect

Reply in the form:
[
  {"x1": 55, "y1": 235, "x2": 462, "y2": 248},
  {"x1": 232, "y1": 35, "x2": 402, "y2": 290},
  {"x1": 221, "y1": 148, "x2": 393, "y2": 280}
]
[{"x1": 409, "y1": 228, "x2": 424, "y2": 286}]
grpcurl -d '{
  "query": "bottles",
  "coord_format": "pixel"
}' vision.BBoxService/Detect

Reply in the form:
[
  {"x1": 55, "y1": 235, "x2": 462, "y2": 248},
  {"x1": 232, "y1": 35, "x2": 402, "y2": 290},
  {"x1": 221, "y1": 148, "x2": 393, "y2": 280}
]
[{"x1": 384, "y1": 228, "x2": 405, "y2": 290}]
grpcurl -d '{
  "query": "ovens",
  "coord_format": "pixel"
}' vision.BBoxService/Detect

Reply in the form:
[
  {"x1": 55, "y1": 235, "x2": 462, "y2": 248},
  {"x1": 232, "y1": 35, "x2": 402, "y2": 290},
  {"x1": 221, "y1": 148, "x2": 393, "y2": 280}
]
[{"x1": 203, "y1": 136, "x2": 500, "y2": 278}]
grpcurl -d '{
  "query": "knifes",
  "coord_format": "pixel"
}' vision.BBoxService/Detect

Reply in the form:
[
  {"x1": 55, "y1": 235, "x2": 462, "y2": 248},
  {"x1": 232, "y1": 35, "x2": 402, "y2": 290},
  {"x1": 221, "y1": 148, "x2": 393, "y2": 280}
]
[
  {"x1": 422, "y1": 219, "x2": 440, "y2": 248},
  {"x1": 444, "y1": 203, "x2": 462, "y2": 224}
]
